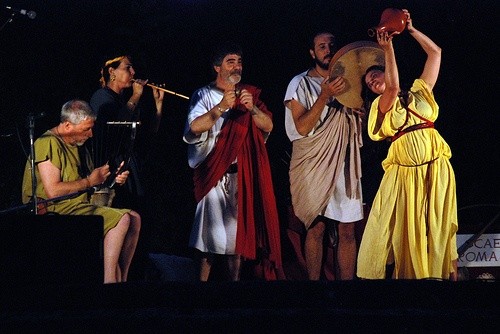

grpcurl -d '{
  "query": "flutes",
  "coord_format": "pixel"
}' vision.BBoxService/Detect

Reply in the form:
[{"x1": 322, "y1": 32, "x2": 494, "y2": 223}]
[{"x1": 130, "y1": 79, "x2": 190, "y2": 99}]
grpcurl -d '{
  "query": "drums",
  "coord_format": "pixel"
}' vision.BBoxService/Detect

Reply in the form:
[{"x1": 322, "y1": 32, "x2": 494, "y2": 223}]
[{"x1": 328, "y1": 40, "x2": 387, "y2": 112}]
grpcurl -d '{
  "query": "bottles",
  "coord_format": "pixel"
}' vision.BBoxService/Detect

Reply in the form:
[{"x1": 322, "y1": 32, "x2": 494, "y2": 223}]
[{"x1": 367, "y1": 8, "x2": 408, "y2": 37}]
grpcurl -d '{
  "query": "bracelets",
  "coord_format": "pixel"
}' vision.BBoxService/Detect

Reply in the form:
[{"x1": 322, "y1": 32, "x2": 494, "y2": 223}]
[
  {"x1": 86, "y1": 174, "x2": 91, "y2": 186},
  {"x1": 217, "y1": 102, "x2": 229, "y2": 112},
  {"x1": 128, "y1": 100, "x2": 136, "y2": 106}
]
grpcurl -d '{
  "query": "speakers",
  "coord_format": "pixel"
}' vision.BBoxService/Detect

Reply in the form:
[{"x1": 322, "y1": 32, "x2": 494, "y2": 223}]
[{"x1": 0, "y1": 214, "x2": 104, "y2": 311}]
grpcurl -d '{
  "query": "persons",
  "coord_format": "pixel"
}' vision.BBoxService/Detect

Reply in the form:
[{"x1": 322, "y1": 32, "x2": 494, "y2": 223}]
[
  {"x1": 180, "y1": 9, "x2": 458, "y2": 282},
  {"x1": 22, "y1": 100, "x2": 142, "y2": 282},
  {"x1": 93, "y1": 53, "x2": 167, "y2": 258}
]
